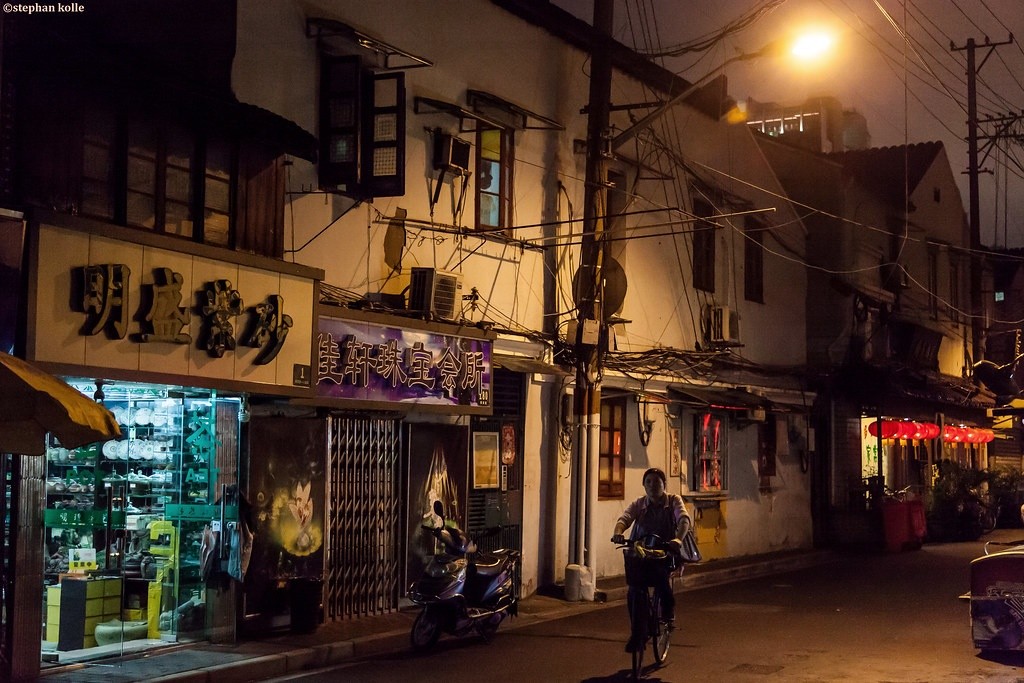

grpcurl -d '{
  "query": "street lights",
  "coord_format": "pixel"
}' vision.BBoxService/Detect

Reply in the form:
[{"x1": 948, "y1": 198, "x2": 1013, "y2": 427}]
[{"x1": 569, "y1": 23, "x2": 840, "y2": 590}]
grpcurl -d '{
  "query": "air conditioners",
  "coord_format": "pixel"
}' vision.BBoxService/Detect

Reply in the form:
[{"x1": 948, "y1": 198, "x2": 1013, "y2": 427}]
[
  {"x1": 437, "y1": 135, "x2": 472, "y2": 177},
  {"x1": 407, "y1": 266, "x2": 465, "y2": 324},
  {"x1": 710, "y1": 305, "x2": 743, "y2": 344}
]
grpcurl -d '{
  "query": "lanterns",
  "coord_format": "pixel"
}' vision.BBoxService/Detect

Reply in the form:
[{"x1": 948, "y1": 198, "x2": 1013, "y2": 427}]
[
  {"x1": 943, "y1": 425, "x2": 995, "y2": 448},
  {"x1": 868, "y1": 419, "x2": 940, "y2": 447}
]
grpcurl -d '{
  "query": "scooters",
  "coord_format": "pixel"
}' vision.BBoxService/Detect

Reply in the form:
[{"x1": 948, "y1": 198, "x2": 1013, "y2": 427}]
[{"x1": 404, "y1": 499, "x2": 522, "y2": 653}]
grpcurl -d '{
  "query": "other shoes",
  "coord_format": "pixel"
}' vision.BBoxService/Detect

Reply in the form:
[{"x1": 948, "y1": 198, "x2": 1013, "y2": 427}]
[
  {"x1": 661, "y1": 610, "x2": 676, "y2": 631},
  {"x1": 625, "y1": 633, "x2": 645, "y2": 653}
]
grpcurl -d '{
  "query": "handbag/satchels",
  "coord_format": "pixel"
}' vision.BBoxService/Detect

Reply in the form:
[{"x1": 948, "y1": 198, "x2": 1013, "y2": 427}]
[{"x1": 667, "y1": 494, "x2": 702, "y2": 563}]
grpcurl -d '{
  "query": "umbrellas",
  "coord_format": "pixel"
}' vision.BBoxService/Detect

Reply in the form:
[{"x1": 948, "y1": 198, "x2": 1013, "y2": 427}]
[{"x1": 0, "y1": 350, "x2": 122, "y2": 455}]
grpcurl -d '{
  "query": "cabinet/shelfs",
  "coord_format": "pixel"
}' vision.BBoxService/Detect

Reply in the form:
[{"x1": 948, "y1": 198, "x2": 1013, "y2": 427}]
[{"x1": 43, "y1": 398, "x2": 213, "y2": 654}]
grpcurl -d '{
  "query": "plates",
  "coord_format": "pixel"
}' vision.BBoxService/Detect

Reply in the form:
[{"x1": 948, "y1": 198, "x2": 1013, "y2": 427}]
[
  {"x1": 102, "y1": 440, "x2": 119, "y2": 460},
  {"x1": 129, "y1": 439, "x2": 144, "y2": 459},
  {"x1": 141, "y1": 442, "x2": 160, "y2": 459},
  {"x1": 116, "y1": 440, "x2": 131, "y2": 460},
  {"x1": 109, "y1": 406, "x2": 169, "y2": 427}
]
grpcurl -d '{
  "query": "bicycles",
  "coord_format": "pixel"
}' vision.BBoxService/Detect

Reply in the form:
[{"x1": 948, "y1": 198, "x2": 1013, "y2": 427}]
[{"x1": 607, "y1": 530, "x2": 679, "y2": 683}]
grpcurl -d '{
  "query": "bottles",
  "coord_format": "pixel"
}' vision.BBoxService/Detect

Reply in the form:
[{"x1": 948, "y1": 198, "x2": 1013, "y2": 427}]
[{"x1": 109, "y1": 469, "x2": 172, "y2": 495}]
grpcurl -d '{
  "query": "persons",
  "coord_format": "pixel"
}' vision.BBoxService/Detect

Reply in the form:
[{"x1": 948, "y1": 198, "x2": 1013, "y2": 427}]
[{"x1": 614, "y1": 468, "x2": 690, "y2": 652}]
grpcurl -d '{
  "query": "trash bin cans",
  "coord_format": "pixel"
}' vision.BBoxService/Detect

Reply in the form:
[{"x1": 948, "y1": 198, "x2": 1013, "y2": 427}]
[{"x1": 288, "y1": 573, "x2": 326, "y2": 635}]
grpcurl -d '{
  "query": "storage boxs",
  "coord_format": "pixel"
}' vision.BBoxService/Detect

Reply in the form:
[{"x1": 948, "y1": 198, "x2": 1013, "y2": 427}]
[{"x1": 46, "y1": 575, "x2": 148, "y2": 649}]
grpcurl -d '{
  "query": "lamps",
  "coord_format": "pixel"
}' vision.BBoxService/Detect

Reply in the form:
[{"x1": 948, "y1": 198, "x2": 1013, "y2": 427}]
[{"x1": 971, "y1": 352, "x2": 1024, "y2": 403}]
